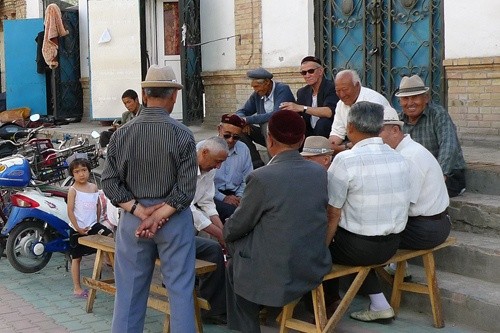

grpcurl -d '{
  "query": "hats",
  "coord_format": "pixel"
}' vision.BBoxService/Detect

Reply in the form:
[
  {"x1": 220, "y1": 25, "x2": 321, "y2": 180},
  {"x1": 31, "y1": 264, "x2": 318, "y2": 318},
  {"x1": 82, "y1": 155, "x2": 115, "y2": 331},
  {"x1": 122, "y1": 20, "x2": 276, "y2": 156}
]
[
  {"x1": 221, "y1": 113, "x2": 245, "y2": 127},
  {"x1": 383, "y1": 106, "x2": 404, "y2": 126},
  {"x1": 141, "y1": 64, "x2": 184, "y2": 90},
  {"x1": 301, "y1": 56, "x2": 321, "y2": 65},
  {"x1": 268, "y1": 110, "x2": 306, "y2": 145},
  {"x1": 299, "y1": 136, "x2": 334, "y2": 157},
  {"x1": 395, "y1": 75, "x2": 429, "y2": 97},
  {"x1": 247, "y1": 67, "x2": 273, "y2": 79}
]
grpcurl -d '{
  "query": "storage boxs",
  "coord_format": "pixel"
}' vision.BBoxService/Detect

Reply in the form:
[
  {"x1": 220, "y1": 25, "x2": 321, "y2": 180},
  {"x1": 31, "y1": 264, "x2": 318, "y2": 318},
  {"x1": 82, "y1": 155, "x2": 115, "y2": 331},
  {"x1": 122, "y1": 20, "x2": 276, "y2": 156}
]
[{"x1": 0, "y1": 155, "x2": 32, "y2": 186}]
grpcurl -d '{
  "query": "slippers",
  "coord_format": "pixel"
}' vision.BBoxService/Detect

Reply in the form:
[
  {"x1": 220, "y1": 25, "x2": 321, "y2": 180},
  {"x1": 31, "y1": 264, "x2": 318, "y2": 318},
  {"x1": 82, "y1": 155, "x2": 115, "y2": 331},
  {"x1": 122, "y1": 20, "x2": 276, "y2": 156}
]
[
  {"x1": 106, "y1": 264, "x2": 113, "y2": 269},
  {"x1": 74, "y1": 289, "x2": 90, "y2": 299}
]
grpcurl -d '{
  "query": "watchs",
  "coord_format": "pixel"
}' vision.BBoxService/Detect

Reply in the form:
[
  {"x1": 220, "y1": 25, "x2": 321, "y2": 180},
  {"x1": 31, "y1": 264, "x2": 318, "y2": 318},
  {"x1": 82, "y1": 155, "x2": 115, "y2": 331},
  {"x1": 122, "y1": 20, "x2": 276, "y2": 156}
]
[
  {"x1": 345, "y1": 143, "x2": 351, "y2": 150},
  {"x1": 302, "y1": 106, "x2": 307, "y2": 114}
]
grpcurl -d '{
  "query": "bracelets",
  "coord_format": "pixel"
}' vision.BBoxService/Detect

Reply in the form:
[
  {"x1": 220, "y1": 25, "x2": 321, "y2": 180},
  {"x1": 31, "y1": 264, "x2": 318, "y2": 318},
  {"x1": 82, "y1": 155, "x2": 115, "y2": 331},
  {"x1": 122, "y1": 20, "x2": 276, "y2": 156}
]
[{"x1": 129, "y1": 201, "x2": 140, "y2": 215}]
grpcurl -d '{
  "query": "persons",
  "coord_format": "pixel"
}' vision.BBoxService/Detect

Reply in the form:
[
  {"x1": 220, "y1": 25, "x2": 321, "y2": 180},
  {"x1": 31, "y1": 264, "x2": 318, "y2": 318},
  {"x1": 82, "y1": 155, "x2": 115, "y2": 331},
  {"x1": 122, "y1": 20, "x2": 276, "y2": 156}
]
[
  {"x1": 279, "y1": 56, "x2": 339, "y2": 140},
  {"x1": 395, "y1": 77, "x2": 465, "y2": 197},
  {"x1": 99, "y1": 88, "x2": 146, "y2": 148},
  {"x1": 382, "y1": 106, "x2": 451, "y2": 281},
  {"x1": 101, "y1": 66, "x2": 199, "y2": 333},
  {"x1": 213, "y1": 114, "x2": 254, "y2": 225},
  {"x1": 222, "y1": 110, "x2": 327, "y2": 333},
  {"x1": 66, "y1": 153, "x2": 113, "y2": 299},
  {"x1": 233, "y1": 69, "x2": 296, "y2": 170},
  {"x1": 300, "y1": 136, "x2": 335, "y2": 171},
  {"x1": 327, "y1": 102, "x2": 410, "y2": 323},
  {"x1": 328, "y1": 70, "x2": 392, "y2": 149},
  {"x1": 189, "y1": 136, "x2": 227, "y2": 325}
]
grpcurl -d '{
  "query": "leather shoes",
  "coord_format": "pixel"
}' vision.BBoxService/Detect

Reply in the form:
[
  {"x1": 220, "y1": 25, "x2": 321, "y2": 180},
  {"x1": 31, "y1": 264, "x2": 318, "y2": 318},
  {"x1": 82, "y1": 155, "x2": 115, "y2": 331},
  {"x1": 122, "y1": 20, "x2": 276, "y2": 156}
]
[
  {"x1": 383, "y1": 264, "x2": 412, "y2": 282},
  {"x1": 350, "y1": 303, "x2": 396, "y2": 324}
]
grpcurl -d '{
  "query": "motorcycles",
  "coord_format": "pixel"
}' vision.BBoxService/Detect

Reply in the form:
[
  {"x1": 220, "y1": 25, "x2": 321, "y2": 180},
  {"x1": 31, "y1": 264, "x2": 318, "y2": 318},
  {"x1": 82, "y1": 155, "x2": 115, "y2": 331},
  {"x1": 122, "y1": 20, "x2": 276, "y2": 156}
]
[
  {"x1": 0, "y1": 114, "x2": 44, "y2": 159},
  {"x1": 0, "y1": 154, "x2": 123, "y2": 273}
]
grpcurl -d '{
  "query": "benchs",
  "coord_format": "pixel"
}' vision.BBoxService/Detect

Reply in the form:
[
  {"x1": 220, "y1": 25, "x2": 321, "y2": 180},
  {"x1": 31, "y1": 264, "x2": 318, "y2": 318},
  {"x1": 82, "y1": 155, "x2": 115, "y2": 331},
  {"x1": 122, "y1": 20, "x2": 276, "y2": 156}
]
[
  {"x1": 276, "y1": 238, "x2": 456, "y2": 333},
  {"x1": 78, "y1": 235, "x2": 218, "y2": 333}
]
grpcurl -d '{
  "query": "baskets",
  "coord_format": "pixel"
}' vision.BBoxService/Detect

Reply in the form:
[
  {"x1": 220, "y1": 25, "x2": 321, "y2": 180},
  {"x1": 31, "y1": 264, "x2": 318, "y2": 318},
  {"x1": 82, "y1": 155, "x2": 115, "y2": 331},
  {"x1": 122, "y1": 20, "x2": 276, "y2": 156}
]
[
  {"x1": 31, "y1": 155, "x2": 66, "y2": 185},
  {"x1": 63, "y1": 144, "x2": 99, "y2": 176}
]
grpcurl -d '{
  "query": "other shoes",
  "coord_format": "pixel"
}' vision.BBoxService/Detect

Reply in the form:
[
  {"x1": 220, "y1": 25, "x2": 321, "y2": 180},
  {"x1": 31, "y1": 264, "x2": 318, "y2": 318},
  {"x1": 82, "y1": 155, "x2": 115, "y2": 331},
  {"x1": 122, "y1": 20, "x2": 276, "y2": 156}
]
[
  {"x1": 313, "y1": 298, "x2": 342, "y2": 326},
  {"x1": 202, "y1": 313, "x2": 227, "y2": 324}
]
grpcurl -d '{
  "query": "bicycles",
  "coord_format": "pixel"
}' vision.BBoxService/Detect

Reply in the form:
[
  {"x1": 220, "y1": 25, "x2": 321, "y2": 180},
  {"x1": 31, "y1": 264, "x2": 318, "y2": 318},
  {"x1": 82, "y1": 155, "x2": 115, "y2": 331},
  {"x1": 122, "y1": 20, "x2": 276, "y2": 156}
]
[{"x1": 0, "y1": 125, "x2": 103, "y2": 257}]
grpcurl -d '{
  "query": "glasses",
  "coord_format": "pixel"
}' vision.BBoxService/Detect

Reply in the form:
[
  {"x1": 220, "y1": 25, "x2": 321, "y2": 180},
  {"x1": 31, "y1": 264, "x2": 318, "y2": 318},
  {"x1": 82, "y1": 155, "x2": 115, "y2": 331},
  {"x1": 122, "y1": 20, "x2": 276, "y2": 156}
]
[
  {"x1": 300, "y1": 67, "x2": 320, "y2": 75},
  {"x1": 221, "y1": 130, "x2": 241, "y2": 140}
]
